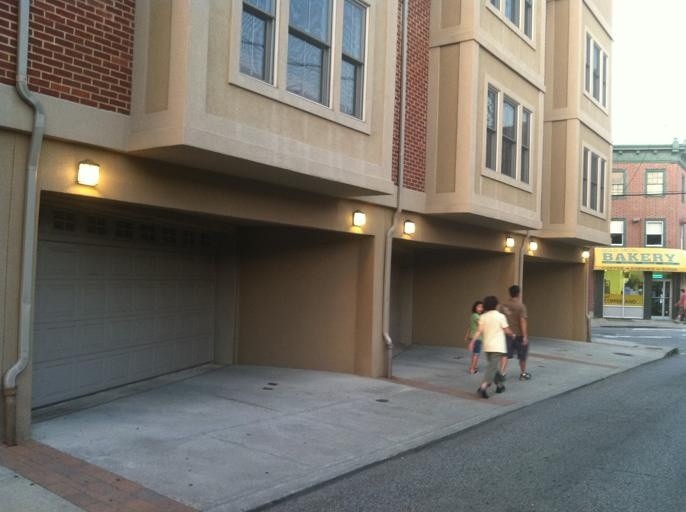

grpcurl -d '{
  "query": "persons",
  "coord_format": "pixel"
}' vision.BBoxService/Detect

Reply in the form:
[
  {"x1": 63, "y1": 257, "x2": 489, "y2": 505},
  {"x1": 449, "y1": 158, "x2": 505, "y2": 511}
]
[
  {"x1": 463, "y1": 300, "x2": 484, "y2": 376},
  {"x1": 467, "y1": 295, "x2": 516, "y2": 399},
  {"x1": 499, "y1": 285, "x2": 531, "y2": 381},
  {"x1": 674, "y1": 289, "x2": 686, "y2": 323}
]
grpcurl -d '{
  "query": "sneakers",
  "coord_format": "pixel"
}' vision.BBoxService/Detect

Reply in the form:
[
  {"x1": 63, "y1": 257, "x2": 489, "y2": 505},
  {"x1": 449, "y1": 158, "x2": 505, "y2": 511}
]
[
  {"x1": 520, "y1": 373, "x2": 532, "y2": 380},
  {"x1": 497, "y1": 386, "x2": 505, "y2": 392},
  {"x1": 477, "y1": 388, "x2": 489, "y2": 398}
]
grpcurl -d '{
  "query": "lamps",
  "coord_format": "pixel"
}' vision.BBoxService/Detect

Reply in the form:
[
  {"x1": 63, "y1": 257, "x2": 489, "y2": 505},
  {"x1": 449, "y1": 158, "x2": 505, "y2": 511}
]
[
  {"x1": 76, "y1": 157, "x2": 101, "y2": 187},
  {"x1": 352, "y1": 208, "x2": 366, "y2": 227},
  {"x1": 529, "y1": 239, "x2": 538, "y2": 251},
  {"x1": 403, "y1": 220, "x2": 416, "y2": 235},
  {"x1": 578, "y1": 248, "x2": 589, "y2": 264},
  {"x1": 505, "y1": 233, "x2": 514, "y2": 247}
]
